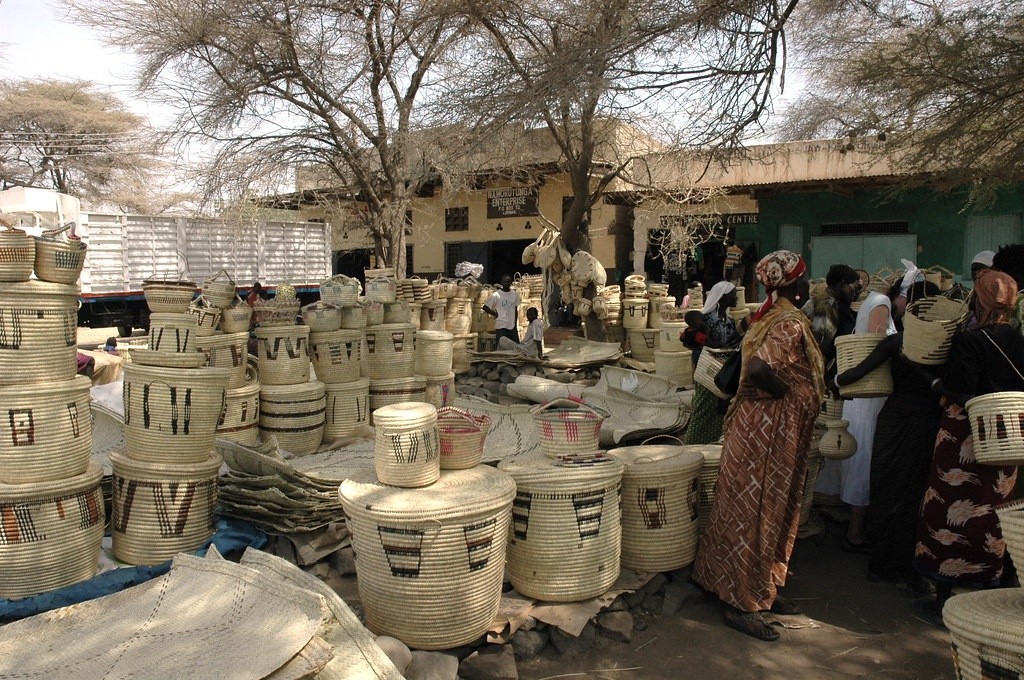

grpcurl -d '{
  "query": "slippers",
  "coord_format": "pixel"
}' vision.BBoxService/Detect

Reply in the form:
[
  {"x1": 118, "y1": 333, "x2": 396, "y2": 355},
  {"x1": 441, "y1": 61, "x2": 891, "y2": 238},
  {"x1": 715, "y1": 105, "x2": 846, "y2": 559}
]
[
  {"x1": 724, "y1": 605, "x2": 781, "y2": 641},
  {"x1": 770, "y1": 594, "x2": 803, "y2": 616},
  {"x1": 845, "y1": 521, "x2": 870, "y2": 547}
]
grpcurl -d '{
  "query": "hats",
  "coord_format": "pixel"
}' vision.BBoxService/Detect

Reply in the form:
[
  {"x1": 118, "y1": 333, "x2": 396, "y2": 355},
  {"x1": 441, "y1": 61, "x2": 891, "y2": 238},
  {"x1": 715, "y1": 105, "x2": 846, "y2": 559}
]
[
  {"x1": 974, "y1": 270, "x2": 1019, "y2": 312},
  {"x1": 826, "y1": 264, "x2": 861, "y2": 291},
  {"x1": 970, "y1": 250, "x2": 996, "y2": 268}
]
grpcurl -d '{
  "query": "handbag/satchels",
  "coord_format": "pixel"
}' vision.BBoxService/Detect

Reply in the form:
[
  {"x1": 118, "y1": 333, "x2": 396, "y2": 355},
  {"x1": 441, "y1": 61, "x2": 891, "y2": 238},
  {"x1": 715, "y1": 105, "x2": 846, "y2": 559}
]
[
  {"x1": 692, "y1": 346, "x2": 739, "y2": 401},
  {"x1": 714, "y1": 349, "x2": 742, "y2": 396}
]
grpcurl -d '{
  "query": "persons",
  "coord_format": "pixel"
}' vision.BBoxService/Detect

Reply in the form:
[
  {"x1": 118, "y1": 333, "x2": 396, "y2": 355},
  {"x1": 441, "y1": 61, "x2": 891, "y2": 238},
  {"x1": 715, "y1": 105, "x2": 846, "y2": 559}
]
[
  {"x1": 104, "y1": 336, "x2": 119, "y2": 356},
  {"x1": 642, "y1": 229, "x2": 1024, "y2": 640},
  {"x1": 77, "y1": 350, "x2": 94, "y2": 378},
  {"x1": 246, "y1": 283, "x2": 267, "y2": 339},
  {"x1": 483, "y1": 272, "x2": 522, "y2": 344},
  {"x1": 497, "y1": 306, "x2": 550, "y2": 361}
]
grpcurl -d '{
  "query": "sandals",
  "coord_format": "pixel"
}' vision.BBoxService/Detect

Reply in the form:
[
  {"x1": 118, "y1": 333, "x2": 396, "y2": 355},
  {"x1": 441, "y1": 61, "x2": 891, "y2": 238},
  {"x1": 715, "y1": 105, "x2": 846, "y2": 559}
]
[
  {"x1": 918, "y1": 593, "x2": 946, "y2": 627},
  {"x1": 867, "y1": 560, "x2": 907, "y2": 585}
]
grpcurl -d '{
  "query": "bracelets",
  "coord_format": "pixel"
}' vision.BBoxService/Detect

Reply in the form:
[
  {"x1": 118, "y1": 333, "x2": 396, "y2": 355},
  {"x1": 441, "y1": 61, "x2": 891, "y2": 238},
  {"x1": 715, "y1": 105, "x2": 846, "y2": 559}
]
[
  {"x1": 932, "y1": 377, "x2": 943, "y2": 389},
  {"x1": 834, "y1": 374, "x2": 840, "y2": 387}
]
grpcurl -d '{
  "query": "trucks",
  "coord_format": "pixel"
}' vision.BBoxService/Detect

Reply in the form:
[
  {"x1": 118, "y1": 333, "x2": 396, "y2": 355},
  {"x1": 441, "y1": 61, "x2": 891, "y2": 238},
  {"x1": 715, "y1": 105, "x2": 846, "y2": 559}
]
[{"x1": 0, "y1": 185, "x2": 334, "y2": 337}]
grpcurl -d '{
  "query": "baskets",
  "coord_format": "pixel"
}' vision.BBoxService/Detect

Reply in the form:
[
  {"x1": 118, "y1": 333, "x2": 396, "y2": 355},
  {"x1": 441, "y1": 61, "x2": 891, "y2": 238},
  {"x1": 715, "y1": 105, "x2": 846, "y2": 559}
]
[
  {"x1": 996, "y1": 499, "x2": 1024, "y2": 590},
  {"x1": 148, "y1": 229, "x2": 669, "y2": 332},
  {"x1": 34, "y1": 222, "x2": 86, "y2": 284},
  {"x1": 834, "y1": 260, "x2": 974, "y2": 401},
  {"x1": 435, "y1": 407, "x2": 491, "y2": 469},
  {"x1": 531, "y1": 397, "x2": 610, "y2": 457},
  {"x1": 0, "y1": 218, "x2": 36, "y2": 282},
  {"x1": 966, "y1": 391, "x2": 1024, "y2": 462}
]
